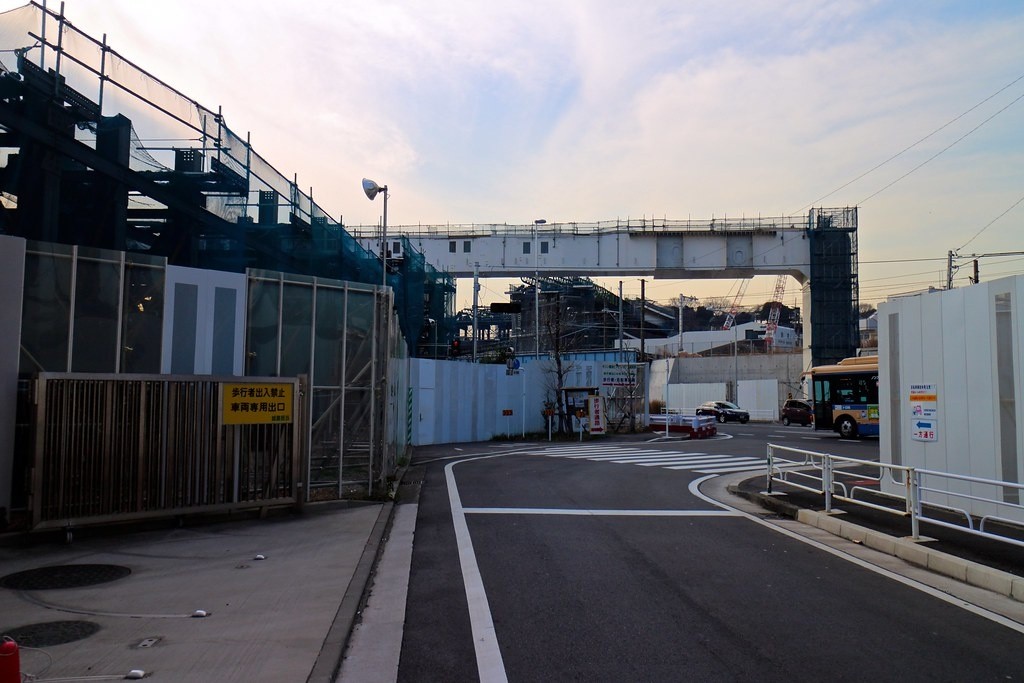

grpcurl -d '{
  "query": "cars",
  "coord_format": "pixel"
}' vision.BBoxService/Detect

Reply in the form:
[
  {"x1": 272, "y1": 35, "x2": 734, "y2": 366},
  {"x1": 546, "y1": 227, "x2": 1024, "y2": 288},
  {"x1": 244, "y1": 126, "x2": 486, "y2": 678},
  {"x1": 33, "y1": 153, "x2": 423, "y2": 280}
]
[
  {"x1": 695, "y1": 401, "x2": 750, "y2": 424},
  {"x1": 782, "y1": 399, "x2": 813, "y2": 427}
]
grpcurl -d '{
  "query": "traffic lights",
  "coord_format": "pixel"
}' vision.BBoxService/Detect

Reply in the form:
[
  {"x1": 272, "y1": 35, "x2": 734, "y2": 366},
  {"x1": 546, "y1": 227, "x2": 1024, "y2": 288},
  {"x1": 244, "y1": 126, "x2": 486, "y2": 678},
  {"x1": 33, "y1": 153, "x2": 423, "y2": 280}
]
[{"x1": 450, "y1": 340, "x2": 461, "y2": 357}]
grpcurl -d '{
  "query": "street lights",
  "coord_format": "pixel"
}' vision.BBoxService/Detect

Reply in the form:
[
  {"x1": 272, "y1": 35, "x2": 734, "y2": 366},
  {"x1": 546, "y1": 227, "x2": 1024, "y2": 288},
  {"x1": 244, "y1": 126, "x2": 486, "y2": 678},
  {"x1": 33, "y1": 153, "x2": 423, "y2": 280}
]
[
  {"x1": 362, "y1": 178, "x2": 389, "y2": 286},
  {"x1": 534, "y1": 218, "x2": 546, "y2": 359}
]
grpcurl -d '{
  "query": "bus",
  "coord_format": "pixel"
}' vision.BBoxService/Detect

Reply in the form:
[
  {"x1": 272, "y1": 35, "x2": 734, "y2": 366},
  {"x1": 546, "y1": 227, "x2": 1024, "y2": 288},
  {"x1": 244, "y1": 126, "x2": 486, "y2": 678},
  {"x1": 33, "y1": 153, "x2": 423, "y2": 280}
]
[{"x1": 797, "y1": 354, "x2": 880, "y2": 440}]
